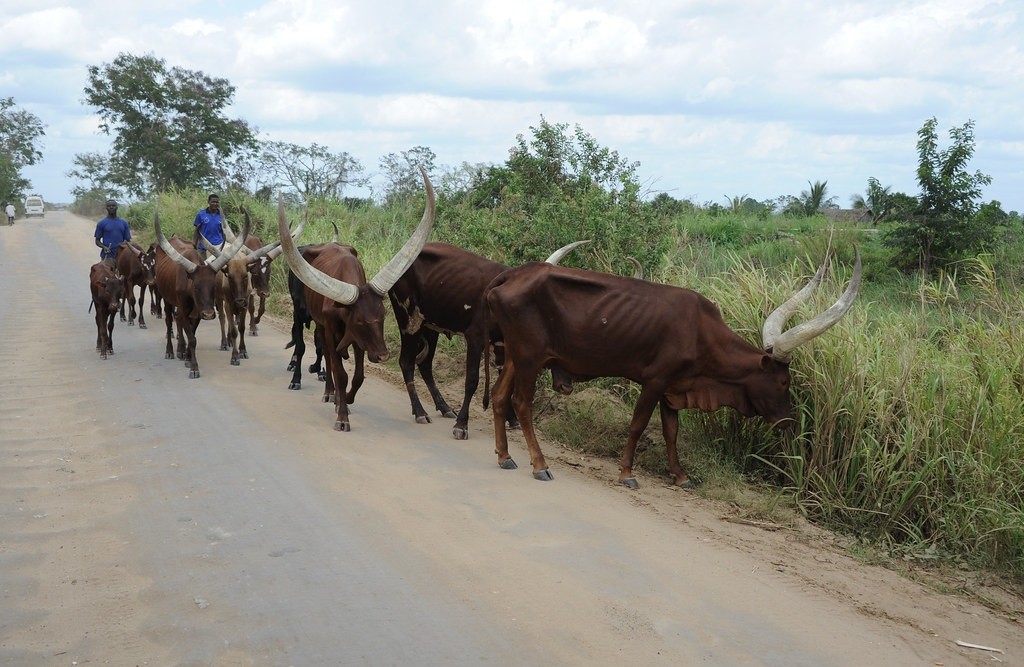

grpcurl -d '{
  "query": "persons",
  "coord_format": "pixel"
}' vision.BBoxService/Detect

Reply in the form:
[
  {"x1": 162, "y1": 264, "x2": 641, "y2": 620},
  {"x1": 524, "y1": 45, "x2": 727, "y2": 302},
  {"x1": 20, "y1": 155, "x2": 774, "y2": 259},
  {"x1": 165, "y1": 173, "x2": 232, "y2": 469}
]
[
  {"x1": 193, "y1": 194, "x2": 226, "y2": 263},
  {"x1": 5, "y1": 202, "x2": 16, "y2": 224},
  {"x1": 94, "y1": 199, "x2": 132, "y2": 260}
]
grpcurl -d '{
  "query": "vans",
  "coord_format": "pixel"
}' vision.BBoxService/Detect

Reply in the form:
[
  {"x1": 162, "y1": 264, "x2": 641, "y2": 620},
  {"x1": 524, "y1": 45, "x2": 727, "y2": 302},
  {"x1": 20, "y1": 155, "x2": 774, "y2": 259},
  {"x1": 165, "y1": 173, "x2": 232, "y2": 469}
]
[{"x1": 25, "y1": 196, "x2": 44, "y2": 218}]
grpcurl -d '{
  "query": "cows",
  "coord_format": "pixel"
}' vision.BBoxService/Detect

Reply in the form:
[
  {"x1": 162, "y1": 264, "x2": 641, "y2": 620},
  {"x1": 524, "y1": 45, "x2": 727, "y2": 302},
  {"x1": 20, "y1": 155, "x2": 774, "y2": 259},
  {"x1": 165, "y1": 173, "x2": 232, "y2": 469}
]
[
  {"x1": 385, "y1": 240, "x2": 596, "y2": 441},
  {"x1": 278, "y1": 163, "x2": 436, "y2": 433},
  {"x1": 482, "y1": 242, "x2": 861, "y2": 491},
  {"x1": 88, "y1": 203, "x2": 308, "y2": 380}
]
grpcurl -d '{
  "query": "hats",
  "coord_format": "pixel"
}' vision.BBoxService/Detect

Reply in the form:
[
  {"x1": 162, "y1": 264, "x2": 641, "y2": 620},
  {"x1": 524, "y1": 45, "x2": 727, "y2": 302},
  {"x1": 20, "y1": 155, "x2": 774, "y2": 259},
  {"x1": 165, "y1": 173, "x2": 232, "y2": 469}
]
[{"x1": 6, "y1": 202, "x2": 12, "y2": 205}]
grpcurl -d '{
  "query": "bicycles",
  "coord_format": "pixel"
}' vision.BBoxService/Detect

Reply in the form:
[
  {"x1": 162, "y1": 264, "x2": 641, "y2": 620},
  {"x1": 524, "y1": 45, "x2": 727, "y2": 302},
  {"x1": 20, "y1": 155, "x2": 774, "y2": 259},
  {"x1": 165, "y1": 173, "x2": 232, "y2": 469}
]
[{"x1": 8, "y1": 217, "x2": 13, "y2": 226}]
